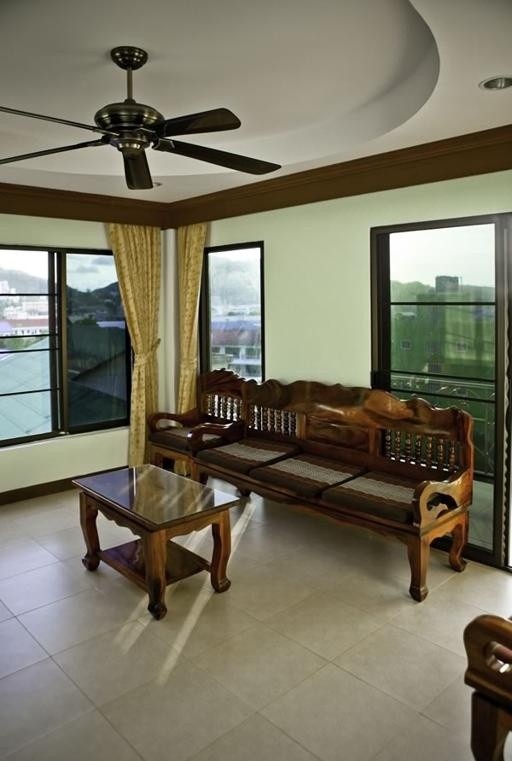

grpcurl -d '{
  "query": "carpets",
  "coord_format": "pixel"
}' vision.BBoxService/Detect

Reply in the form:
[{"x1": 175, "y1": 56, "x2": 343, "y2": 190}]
[{"x1": 100, "y1": 538, "x2": 211, "y2": 594}]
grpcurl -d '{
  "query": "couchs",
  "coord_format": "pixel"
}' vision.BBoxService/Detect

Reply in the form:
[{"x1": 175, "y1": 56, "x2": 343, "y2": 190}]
[{"x1": 189, "y1": 369, "x2": 474, "y2": 602}]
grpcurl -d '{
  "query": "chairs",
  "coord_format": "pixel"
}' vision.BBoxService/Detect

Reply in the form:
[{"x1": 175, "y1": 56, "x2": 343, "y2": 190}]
[
  {"x1": 146, "y1": 368, "x2": 245, "y2": 482},
  {"x1": 464, "y1": 613, "x2": 512, "y2": 761}
]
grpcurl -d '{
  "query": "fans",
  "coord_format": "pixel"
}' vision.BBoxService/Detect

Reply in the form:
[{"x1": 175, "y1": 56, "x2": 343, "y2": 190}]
[{"x1": 0, "y1": 46, "x2": 282, "y2": 190}]
